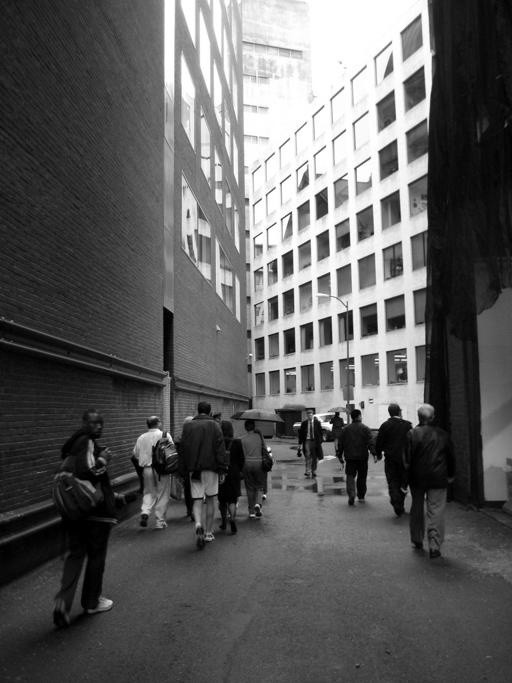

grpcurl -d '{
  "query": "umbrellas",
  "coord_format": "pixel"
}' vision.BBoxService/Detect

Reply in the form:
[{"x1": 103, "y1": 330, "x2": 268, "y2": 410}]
[{"x1": 229, "y1": 407, "x2": 287, "y2": 431}]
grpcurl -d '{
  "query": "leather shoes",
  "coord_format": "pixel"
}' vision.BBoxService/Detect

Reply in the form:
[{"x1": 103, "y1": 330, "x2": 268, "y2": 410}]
[{"x1": 429, "y1": 549, "x2": 441, "y2": 558}]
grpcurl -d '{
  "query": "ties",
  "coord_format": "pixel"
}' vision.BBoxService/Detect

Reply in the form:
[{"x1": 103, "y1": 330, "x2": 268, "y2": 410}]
[{"x1": 309, "y1": 420, "x2": 315, "y2": 442}]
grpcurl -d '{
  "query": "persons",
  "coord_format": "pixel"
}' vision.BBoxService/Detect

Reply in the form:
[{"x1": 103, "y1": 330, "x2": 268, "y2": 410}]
[
  {"x1": 171, "y1": 415, "x2": 200, "y2": 521},
  {"x1": 238, "y1": 419, "x2": 272, "y2": 519},
  {"x1": 297, "y1": 408, "x2": 325, "y2": 475},
  {"x1": 254, "y1": 427, "x2": 274, "y2": 498},
  {"x1": 400, "y1": 402, "x2": 457, "y2": 558},
  {"x1": 181, "y1": 399, "x2": 229, "y2": 548},
  {"x1": 330, "y1": 411, "x2": 344, "y2": 448},
  {"x1": 335, "y1": 407, "x2": 377, "y2": 505},
  {"x1": 216, "y1": 418, "x2": 246, "y2": 534},
  {"x1": 373, "y1": 402, "x2": 412, "y2": 518},
  {"x1": 131, "y1": 414, "x2": 176, "y2": 530},
  {"x1": 52, "y1": 410, "x2": 119, "y2": 630}
]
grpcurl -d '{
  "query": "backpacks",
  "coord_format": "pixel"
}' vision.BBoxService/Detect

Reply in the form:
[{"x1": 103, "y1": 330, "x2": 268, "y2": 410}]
[
  {"x1": 155, "y1": 429, "x2": 181, "y2": 477},
  {"x1": 53, "y1": 455, "x2": 104, "y2": 522},
  {"x1": 259, "y1": 446, "x2": 275, "y2": 471}
]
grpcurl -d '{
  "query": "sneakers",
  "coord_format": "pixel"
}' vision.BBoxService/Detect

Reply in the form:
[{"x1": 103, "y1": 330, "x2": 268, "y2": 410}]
[
  {"x1": 202, "y1": 531, "x2": 216, "y2": 543},
  {"x1": 52, "y1": 599, "x2": 69, "y2": 625},
  {"x1": 86, "y1": 594, "x2": 113, "y2": 615},
  {"x1": 155, "y1": 518, "x2": 167, "y2": 529},
  {"x1": 194, "y1": 524, "x2": 206, "y2": 549},
  {"x1": 254, "y1": 503, "x2": 262, "y2": 517},
  {"x1": 139, "y1": 512, "x2": 150, "y2": 528}
]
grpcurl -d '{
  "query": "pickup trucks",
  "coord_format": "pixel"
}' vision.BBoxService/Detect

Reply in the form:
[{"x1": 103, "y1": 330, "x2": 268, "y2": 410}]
[{"x1": 292, "y1": 412, "x2": 348, "y2": 442}]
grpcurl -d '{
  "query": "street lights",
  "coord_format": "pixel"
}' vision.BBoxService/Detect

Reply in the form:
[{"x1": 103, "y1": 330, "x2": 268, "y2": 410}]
[{"x1": 315, "y1": 292, "x2": 353, "y2": 426}]
